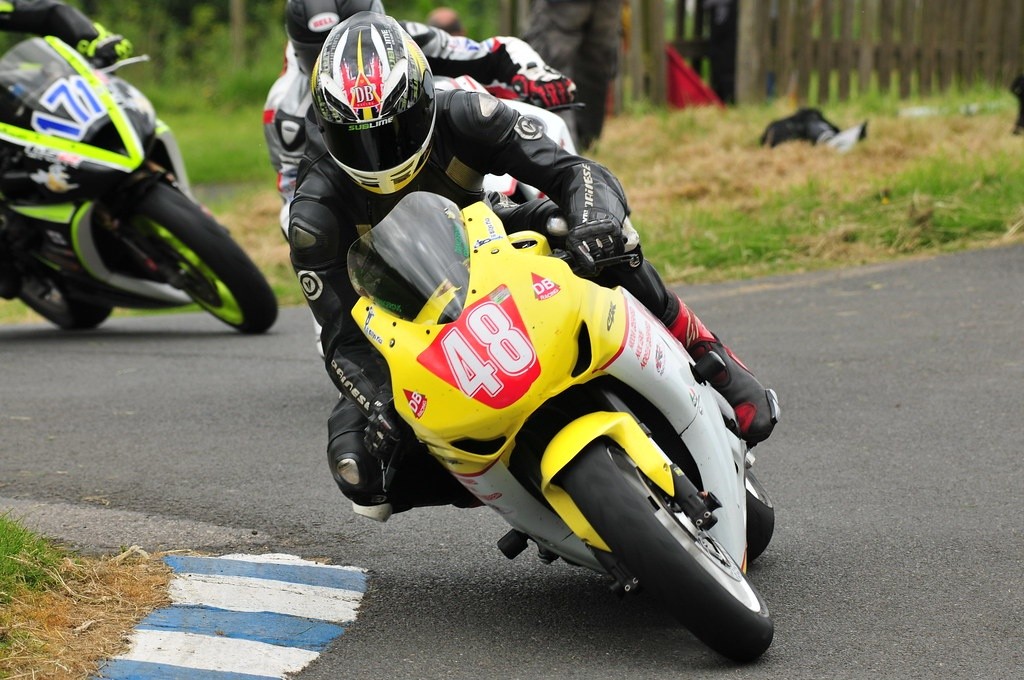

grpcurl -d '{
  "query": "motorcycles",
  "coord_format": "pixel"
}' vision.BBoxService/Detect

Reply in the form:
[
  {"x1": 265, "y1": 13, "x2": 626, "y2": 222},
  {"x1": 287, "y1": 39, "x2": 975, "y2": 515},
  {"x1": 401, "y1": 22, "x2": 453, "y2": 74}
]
[
  {"x1": 337, "y1": 190, "x2": 777, "y2": 662},
  {"x1": 0, "y1": 18, "x2": 280, "y2": 334}
]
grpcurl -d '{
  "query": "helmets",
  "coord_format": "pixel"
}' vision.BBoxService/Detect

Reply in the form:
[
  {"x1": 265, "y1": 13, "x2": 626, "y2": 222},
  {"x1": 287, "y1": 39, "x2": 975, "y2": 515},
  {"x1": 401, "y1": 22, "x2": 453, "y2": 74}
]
[
  {"x1": 309, "y1": 11, "x2": 436, "y2": 197},
  {"x1": 285, "y1": 0, "x2": 387, "y2": 79}
]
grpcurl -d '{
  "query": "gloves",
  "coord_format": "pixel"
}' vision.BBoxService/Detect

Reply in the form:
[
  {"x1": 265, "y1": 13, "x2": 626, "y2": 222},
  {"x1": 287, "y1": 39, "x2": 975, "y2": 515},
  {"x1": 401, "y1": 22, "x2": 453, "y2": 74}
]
[
  {"x1": 567, "y1": 206, "x2": 630, "y2": 277},
  {"x1": 509, "y1": 58, "x2": 578, "y2": 112},
  {"x1": 360, "y1": 391, "x2": 406, "y2": 470},
  {"x1": 76, "y1": 21, "x2": 139, "y2": 73}
]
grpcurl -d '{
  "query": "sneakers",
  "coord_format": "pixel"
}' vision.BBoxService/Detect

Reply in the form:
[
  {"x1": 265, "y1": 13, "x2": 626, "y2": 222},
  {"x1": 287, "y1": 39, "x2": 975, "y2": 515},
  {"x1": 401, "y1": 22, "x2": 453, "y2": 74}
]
[{"x1": 692, "y1": 336, "x2": 782, "y2": 446}]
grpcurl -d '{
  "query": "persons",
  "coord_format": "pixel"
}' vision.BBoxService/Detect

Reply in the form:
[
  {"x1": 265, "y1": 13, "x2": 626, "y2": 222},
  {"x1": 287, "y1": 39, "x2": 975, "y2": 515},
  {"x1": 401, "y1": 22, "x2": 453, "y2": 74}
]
[
  {"x1": 0, "y1": 0, "x2": 132, "y2": 299},
  {"x1": 263, "y1": 0, "x2": 576, "y2": 362},
  {"x1": 427, "y1": 7, "x2": 463, "y2": 40},
  {"x1": 522, "y1": 0, "x2": 618, "y2": 150},
  {"x1": 288, "y1": 11, "x2": 781, "y2": 522}
]
